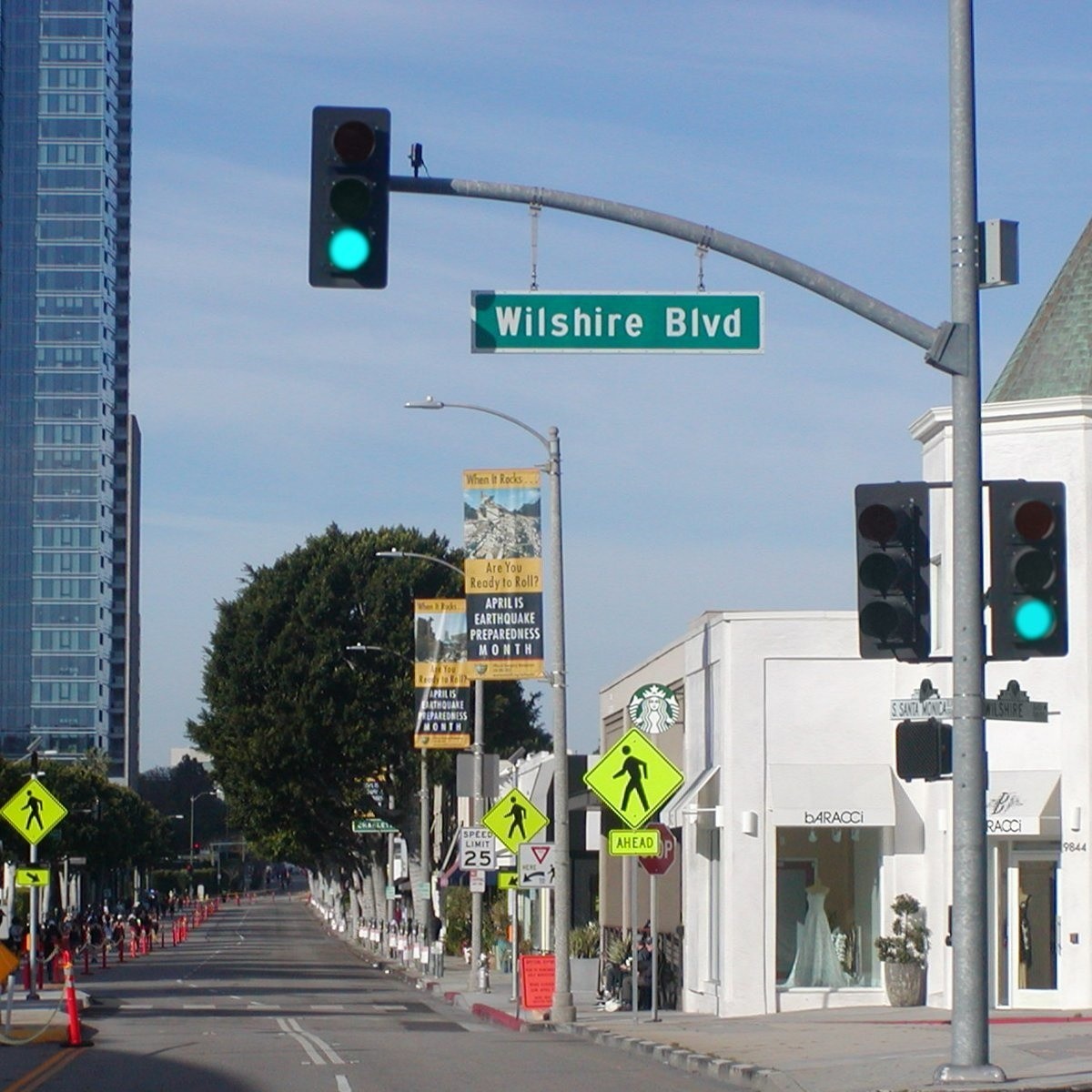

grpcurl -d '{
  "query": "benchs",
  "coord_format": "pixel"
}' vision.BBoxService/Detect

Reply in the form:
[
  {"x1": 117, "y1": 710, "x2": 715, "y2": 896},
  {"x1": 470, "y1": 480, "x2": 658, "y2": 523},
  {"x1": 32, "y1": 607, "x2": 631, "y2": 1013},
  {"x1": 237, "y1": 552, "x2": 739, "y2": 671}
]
[{"x1": 607, "y1": 964, "x2": 668, "y2": 1012}]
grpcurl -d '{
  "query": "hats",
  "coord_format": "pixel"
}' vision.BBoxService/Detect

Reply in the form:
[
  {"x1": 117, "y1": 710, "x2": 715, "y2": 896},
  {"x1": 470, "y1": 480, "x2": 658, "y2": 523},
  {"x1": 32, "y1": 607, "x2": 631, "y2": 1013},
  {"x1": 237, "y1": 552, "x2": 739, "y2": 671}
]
[{"x1": 643, "y1": 936, "x2": 653, "y2": 945}]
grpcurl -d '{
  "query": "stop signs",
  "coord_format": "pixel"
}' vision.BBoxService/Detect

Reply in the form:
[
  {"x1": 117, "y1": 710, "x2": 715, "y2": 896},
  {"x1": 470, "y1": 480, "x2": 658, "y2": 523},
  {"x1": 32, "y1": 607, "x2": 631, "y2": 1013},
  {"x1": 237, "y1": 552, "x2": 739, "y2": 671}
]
[{"x1": 636, "y1": 823, "x2": 677, "y2": 875}]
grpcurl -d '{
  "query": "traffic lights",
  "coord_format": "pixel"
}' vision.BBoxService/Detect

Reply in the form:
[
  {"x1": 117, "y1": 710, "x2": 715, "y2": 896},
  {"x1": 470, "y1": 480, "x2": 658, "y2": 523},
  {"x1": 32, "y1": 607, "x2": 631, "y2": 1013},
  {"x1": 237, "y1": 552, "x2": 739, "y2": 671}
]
[
  {"x1": 193, "y1": 843, "x2": 199, "y2": 849},
  {"x1": 988, "y1": 480, "x2": 1068, "y2": 659},
  {"x1": 187, "y1": 865, "x2": 193, "y2": 873},
  {"x1": 308, "y1": 105, "x2": 391, "y2": 290},
  {"x1": 855, "y1": 480, "x2": 931, "y2": 660},
  {"x1": 896, "y1": 722, "x2": 952, "y2": 778}
]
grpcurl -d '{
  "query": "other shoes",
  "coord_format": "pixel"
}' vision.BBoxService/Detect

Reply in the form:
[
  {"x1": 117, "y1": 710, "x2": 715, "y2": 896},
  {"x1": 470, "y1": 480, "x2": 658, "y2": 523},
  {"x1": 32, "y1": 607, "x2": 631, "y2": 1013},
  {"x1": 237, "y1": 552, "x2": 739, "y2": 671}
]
[
  {"x1": 597, "y1": 996, "x2": 608, "y2": 1001},
  {"x1": 593, "y1": 1003, "x2": 603, "y2": 1009}
]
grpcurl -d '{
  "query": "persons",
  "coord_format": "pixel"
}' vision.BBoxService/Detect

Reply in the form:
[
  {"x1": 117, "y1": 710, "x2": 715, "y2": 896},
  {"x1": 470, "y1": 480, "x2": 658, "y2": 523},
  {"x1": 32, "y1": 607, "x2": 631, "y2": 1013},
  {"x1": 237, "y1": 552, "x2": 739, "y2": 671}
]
[
  {"x1": 785, "y1": 877, "x2": 844, "y2": 987},
  {"x1": 0, "y1": 888, "x2": 192, "y2": 983},
  {"x1": 592, "y1": 935, "x2": 667, "y2": 1012},
  {"x1": 1018, "y1": 888, "x2": 1033, "y2": 989}
]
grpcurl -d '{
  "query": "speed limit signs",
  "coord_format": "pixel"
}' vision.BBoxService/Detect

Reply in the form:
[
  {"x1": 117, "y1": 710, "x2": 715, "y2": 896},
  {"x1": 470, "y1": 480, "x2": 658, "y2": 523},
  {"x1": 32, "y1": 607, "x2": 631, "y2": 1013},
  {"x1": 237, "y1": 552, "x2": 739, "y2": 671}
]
[{"x1": 459, "y1": 827, "x2": 496, "y2": 871}]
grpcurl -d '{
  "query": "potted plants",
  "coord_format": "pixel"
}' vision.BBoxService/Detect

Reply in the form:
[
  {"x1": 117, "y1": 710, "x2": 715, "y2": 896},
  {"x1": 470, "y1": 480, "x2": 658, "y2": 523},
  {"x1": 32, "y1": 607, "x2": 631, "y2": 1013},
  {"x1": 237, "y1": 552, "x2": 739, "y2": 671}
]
[{"x1": 874, "y1": 894, "x2": 929, "y2": 1005}]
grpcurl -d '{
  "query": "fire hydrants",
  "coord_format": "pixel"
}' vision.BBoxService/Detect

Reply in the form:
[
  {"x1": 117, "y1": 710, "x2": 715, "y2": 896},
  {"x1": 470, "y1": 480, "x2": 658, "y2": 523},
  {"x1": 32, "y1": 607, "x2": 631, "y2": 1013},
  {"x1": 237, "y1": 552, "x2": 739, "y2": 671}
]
[{"x1": 477, "y1": 951, "x2": 491, "y2": 993}]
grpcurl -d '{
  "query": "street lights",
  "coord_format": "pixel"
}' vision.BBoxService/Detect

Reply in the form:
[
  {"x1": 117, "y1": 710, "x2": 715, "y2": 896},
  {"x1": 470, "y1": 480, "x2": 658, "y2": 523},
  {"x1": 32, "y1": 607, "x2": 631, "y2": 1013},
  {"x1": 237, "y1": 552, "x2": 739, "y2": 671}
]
[
  {"x1": 145, "y1": 815, "x2": 184, "y2": 890},
  {"x1": 348, "y1": 642, "x2": 436, "y2": 975},
  {"x1": 406, "y1": 395, "x2": 577, "y2": 1022},
  {"x1": 377, "y1": 547, "x2": 484, "y2": 979},
  {"x1": 13, "y1": 737, "x2": 58, "y2": 1000},
  {"x1": 190, "y1": 791, "x2": 216, "y2": 901}
]
